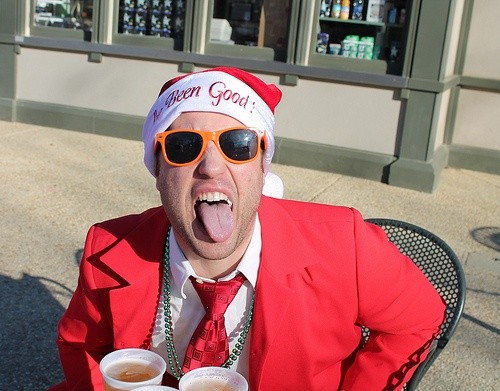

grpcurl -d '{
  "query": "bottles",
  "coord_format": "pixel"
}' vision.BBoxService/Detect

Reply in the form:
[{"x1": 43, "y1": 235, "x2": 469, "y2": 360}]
[
  {"x1": 352, "y1": 0, "x2": 364, "y2": 20},
  {"x1": 320, "y1": 0, "x2": 332, "y2": 18},
  {"x1": 340, "y1": 0, "x2": 351, "y2": 20},
  {"x1": 331, "y1": 0, "x2": 342, "y2": 19},
  {"x1": 388, "y1": 7, "x2": 406, "y2": 25}
]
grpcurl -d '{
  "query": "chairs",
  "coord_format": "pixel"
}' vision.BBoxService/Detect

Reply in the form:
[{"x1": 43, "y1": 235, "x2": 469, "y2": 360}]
[{"x1": 361, "y1": 218, "x2": 466, "y2": 391}]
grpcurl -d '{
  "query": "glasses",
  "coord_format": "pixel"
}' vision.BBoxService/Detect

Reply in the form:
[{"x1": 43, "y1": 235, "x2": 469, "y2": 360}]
[{"x1": 153, "y1": 128, "x2": 268, "y2": 166}]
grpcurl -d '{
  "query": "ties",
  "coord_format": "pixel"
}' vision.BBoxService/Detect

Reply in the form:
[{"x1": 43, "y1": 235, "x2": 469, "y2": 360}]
[{"x1": 180, "y1": 271, "x2": 248, "y2": 378}]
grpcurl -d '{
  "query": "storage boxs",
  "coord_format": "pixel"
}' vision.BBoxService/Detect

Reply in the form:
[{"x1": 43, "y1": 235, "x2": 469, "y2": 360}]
[
  {"x1": 366, "y1": 0, "x2": 386, "y2": 22},
  {"x1": 122, "y1": 0, "x2": 186, "y2": 39}
]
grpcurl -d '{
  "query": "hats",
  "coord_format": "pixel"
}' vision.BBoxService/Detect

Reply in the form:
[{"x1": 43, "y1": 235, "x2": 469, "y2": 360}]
[{"x1": 141, "y1": 66, "x2": 285, "y2": 198}]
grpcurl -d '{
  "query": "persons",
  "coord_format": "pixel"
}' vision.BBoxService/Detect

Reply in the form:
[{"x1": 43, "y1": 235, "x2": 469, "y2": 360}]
[{"x1": 49, "y1": 67, "x2": 446, "y2": 391}]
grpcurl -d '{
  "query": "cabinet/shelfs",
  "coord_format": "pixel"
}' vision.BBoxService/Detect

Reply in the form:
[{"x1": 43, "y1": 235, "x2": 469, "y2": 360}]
[{"x1": 320, "y1": 0, "x2": 409, "y2": 61}]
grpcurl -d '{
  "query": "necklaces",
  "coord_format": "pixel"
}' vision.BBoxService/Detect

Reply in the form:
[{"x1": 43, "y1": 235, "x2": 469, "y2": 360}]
[{"x1": 164, "y1": 224, "x2": 256, "y2": 379}]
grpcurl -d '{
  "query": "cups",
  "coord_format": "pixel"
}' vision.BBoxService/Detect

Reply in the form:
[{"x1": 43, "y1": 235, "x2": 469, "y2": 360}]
[
  {"x1": 178, "y1": 366, "x2": 249, "y2": 391},
  {"x1": 99, "y1": 347, "x2": 167, "y2": 391}
]
